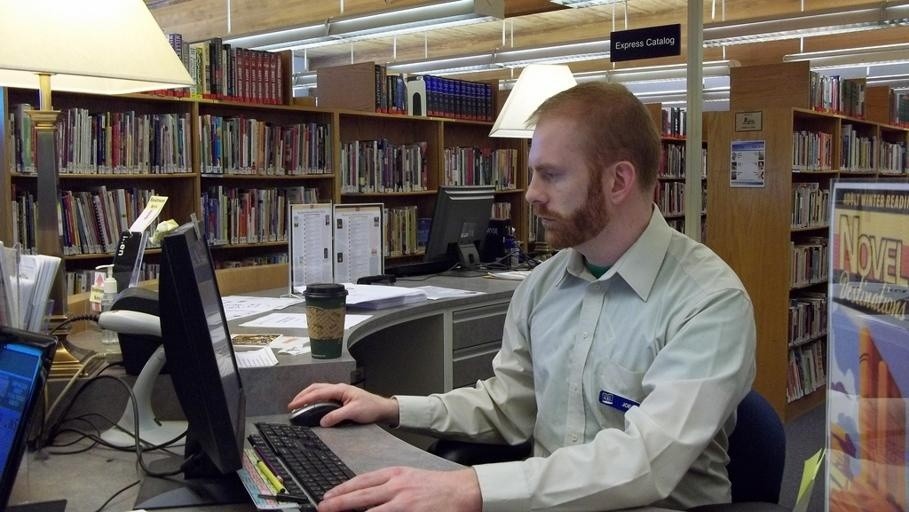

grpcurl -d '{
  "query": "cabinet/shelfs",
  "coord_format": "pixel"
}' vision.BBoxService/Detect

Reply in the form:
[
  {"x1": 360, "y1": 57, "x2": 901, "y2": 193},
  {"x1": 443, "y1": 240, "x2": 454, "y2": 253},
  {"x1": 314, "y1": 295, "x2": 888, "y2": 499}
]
[{"x1": 703, "y1": 60, "x2": 909, "y2": 425}]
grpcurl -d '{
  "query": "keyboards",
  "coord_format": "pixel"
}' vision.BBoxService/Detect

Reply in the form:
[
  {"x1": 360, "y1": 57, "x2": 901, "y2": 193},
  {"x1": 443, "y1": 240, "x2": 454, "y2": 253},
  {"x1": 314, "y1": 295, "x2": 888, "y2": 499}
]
[{"x1": 255, "y1": 423, "x2": 357, "y2": 512}]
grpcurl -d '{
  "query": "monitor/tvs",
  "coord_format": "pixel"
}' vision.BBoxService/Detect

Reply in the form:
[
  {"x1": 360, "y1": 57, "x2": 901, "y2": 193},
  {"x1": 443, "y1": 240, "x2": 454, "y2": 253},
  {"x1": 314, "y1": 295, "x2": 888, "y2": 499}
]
[
  {"x1": 422, "y1": 184, "x2": 496, "y2": 278},
  {"x1": 132, "y1": 220, "x2": 253, "y2": 510},
  {"x1": 0, "y1": 325, "x2": 58, "y2": 512}
]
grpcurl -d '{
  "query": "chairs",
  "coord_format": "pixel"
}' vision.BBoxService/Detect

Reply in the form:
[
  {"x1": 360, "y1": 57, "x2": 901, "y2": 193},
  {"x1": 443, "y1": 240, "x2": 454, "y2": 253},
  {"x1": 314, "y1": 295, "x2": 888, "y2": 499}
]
[{"x1": 426, "y1": 384, "x2": 791, "y2": 512}]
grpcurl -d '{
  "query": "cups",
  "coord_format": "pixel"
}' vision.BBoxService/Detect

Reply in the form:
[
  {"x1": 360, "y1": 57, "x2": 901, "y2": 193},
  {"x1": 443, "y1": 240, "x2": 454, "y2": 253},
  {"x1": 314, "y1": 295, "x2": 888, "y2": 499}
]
[{"x1": 303, "y1": 282, "x2": 348, "y2": 358}]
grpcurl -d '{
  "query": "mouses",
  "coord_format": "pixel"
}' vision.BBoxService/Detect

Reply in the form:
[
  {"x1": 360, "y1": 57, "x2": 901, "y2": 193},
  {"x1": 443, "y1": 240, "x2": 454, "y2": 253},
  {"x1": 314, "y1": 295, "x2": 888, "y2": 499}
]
[{"x1": 288, "y1": 399, "x2": 348, "y2": 427}]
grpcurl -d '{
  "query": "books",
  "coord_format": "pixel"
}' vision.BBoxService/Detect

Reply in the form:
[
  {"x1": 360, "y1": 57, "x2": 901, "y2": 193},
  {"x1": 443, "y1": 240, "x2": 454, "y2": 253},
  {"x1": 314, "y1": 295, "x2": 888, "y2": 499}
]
[
  {"x1": 444, "y1": 145, "x2": 519, "y2": 191},
  {"x1": 794, "y1": 70, "x2": 907, "y2": 173},
  {"x1": 491, "y1": 202, "x2": 511, "y2": 218},
  {"x1": 654, "y1": 105, "x2": 707, "y2": 242},
  {"x1": 384, "y1": 204, "x2": 418, "y2": 257},
  {"x1": 528, "y1": 203, "x2": 550, "y2": 252},
  {"x1": 789, "y1": 292, "x2": 827, "y2": 345},
  {"x1": 201, "y1": 186, "x2": 318, "y2": 245},
  {"x1": 792, "y1": 237, "x2": 828, "y2": 285},
  {"x1": 341, "y1": 140, "x2": 429, "y2": 193},
  {"x1": 788, "y1": 337, "x2": 826, "y2": 403},
  {"x1": 375, "y1": 63, "x2": 492, "y2": 122},
  {"x1": 13, "y1": 193, "x2": 37, "y2": 251},
  {"x1": 58, "y1": 185, "x2": 161, "y2": 257},
  {"x1": 139, "y1": 33, "x2": 284, "y2": 105},
  {"x1": 8, "y1": 104, "x2": 335, "y2": 174},
  {"x1": 791, "y1": 184, "x2": 829, "y2": 229}
]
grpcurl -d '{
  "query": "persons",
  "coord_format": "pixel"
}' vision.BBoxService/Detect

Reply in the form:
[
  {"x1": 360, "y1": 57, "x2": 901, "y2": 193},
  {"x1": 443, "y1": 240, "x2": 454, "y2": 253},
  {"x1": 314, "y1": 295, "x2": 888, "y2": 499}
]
[{"x1": 285, "y1": 81, "x2": 757, "y2": 510}]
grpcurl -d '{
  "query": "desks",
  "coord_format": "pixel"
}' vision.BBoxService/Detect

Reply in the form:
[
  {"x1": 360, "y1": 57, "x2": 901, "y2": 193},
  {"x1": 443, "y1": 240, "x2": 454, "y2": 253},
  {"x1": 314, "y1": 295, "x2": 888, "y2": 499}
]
[{"x1": 0, "y1": 269, "x2": 530, "y2": 512}]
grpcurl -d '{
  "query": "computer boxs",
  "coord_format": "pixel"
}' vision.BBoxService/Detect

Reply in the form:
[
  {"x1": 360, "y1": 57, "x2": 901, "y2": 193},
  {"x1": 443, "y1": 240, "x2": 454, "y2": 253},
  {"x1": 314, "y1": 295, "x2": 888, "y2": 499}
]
[{"x1": 486, "y1": 218, "x2": 512, "y2": 270}]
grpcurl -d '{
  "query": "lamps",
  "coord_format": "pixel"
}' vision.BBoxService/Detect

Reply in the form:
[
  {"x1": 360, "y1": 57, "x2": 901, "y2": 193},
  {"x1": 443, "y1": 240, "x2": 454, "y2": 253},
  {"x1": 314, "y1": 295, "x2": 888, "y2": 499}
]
[
  {"x1": 0, "y1": 0, "x2": 196, "y2": 340},
  {"x1": 486, "y1": 62, "x2": 576, "y2": 142}
]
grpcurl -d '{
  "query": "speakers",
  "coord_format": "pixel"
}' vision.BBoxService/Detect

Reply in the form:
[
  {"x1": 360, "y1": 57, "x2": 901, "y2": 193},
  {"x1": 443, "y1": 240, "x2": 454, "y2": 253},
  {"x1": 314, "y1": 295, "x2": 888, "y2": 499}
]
[{"x1": 110, "y1": 287, "x2": 173, "y2": 376}]
